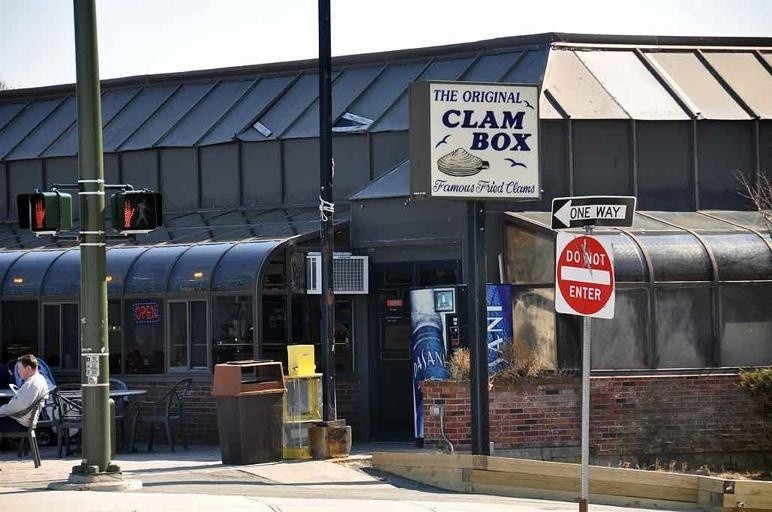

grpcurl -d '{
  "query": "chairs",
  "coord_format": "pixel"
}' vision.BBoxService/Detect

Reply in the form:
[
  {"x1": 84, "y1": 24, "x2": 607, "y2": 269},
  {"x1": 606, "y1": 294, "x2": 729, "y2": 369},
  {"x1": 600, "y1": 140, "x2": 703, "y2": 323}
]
[{"x1": 1, "y1": 376, "x2": 195, "y2": 470}]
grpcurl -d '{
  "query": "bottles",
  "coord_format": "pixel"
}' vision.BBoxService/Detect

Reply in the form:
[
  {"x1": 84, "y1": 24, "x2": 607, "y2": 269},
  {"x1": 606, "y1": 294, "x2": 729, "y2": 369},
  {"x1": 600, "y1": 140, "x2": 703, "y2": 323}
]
[{"x1": 411, "y1": 291, "x2": 452, "y2": 436}]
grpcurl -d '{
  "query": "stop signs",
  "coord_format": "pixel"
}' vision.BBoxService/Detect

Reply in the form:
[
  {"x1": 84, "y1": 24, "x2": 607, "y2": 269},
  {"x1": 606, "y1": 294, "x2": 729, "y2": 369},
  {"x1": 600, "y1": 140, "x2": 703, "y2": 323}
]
[{"x1": 554, "y1": 233, "x2": 613, "y2": 320}]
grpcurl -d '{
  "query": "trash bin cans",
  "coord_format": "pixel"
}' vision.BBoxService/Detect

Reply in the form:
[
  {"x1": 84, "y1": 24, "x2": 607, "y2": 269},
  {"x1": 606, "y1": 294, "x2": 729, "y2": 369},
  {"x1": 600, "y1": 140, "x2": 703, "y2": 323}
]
[{"x1": 211, "y1": 359, "x2": 287, "y2": 465}]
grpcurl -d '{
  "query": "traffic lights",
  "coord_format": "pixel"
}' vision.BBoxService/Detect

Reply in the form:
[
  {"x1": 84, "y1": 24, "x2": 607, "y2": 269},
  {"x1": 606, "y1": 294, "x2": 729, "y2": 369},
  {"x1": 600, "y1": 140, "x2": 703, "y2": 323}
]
[
  {"x1": 17, "y1": 191, "x2": 73, "y2": 232},
  {"x1": 112, "y1": 187, "x2": 163, "y2": 235}
]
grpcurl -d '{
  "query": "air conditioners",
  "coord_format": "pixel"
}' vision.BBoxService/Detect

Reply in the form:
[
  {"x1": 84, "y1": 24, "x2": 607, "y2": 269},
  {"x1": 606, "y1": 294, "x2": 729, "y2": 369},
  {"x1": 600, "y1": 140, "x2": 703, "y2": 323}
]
[{"x1": 307, "y1": 252, "x2": 371, "y2": 296}]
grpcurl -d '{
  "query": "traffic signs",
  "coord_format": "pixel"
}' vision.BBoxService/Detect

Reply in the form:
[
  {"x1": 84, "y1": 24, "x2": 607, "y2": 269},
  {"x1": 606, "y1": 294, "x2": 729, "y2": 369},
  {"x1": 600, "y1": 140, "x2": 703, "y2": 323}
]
[{"x1": 550, "y1": 196, "x2": 636, "y2": 231}]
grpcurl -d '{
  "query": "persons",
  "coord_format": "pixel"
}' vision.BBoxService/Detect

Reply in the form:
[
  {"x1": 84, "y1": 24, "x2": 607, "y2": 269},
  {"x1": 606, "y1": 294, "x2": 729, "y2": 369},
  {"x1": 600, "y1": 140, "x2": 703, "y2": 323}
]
[{"x1": 1, "y1": 353, "x2": 48, "y2": 433}]
